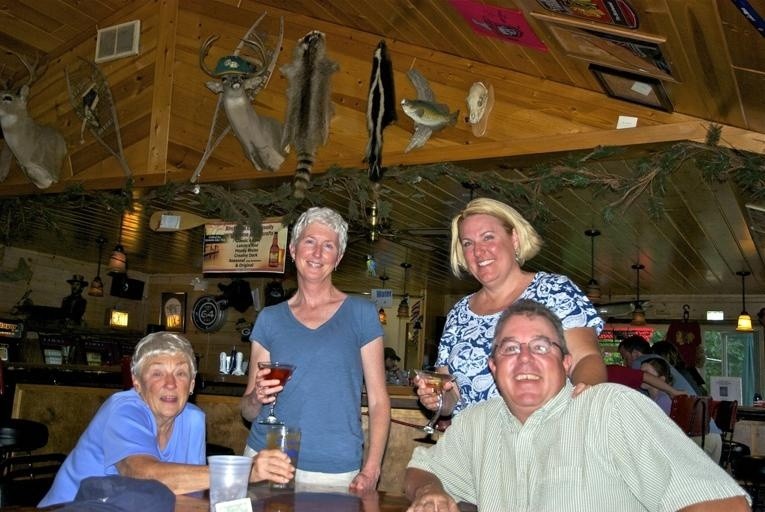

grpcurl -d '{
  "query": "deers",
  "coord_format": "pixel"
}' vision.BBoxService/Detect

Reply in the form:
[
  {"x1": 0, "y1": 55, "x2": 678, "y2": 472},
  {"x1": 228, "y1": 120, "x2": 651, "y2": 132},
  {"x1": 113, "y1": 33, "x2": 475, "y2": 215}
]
[
  {"x1": 198, "y1": 31, "x2": 290, "y2": 172},
  {"x1": 0, "y1": 46, "x2": 67, "y2": 191}
]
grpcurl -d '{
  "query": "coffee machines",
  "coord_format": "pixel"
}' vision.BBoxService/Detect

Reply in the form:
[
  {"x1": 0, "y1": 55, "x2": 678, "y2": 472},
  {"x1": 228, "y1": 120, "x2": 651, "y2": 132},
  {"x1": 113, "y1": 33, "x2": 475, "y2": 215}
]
[{"x1": 0, "y1": 317, "x2": 27, "y2": 365}]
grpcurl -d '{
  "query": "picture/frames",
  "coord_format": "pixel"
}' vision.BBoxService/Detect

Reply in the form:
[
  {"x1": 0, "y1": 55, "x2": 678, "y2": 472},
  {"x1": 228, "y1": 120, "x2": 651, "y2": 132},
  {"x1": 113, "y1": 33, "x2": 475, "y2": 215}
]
[
  {"x1": 159, "y1": 291, "x2": 187, "y2": 334},
  {"x1": 530, "y1": 12, "x2": 684, "y2": 85},
  {"x1": 588, "y1": 63, "x2": 674, "y2": 114}
]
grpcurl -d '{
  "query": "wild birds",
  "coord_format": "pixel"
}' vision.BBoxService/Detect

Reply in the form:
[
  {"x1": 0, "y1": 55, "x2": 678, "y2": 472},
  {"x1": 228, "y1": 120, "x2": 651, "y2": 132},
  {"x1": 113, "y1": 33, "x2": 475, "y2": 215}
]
[{"x1": 404, "y1": 69, "x2": 450, "y2": 154}]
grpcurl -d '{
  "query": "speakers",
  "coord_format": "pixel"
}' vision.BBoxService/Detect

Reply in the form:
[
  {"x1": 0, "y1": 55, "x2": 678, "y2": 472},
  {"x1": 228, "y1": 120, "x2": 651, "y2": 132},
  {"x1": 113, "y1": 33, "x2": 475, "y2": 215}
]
[
  {"x1": 110, "y1": 277, "x2": 145, "y2": 300},
  {"x1": 429, "y1": 316, "x2": 447, "y2": 372}
]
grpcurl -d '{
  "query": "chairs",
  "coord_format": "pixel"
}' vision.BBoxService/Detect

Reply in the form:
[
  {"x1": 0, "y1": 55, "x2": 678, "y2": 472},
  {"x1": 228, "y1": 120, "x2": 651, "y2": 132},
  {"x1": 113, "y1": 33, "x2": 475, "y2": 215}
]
[
  {"x1": 731, "y1": 456, "x2": 765, "y2": 512},
  {"x1": 670, "y1": 394, "x2": 710, "y2": 451},
  {"x1": 0, "y1": 419, "x2": 67, "y2": 508},
  {"x1": 713, "y1": 400, "x2": 737, "y2": 470},
  {"x1": 0, "y1": 453, "x2": 68, "y2": 511}
]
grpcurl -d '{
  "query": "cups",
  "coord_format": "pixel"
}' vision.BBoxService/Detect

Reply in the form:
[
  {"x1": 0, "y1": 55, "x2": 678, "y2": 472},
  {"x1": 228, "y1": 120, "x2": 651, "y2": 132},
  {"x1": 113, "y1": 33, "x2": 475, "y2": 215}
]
[
  {"x1": 264, "y1": 423, "x2": 303, "y2": 493},
  {"x1": 206, "y1": 452, "x2": 256, "y2": 512},
  {"x1": 277, "y1": 241, "x2": 285, "y2": 266}
]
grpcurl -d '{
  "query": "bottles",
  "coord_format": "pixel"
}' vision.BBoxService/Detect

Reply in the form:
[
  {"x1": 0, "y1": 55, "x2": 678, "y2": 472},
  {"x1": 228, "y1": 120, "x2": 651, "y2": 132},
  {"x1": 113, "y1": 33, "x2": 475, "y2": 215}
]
[
  {"x1": 268, "y1": 232, "x2": 280, "y2": 267},
  {"x1": 226, "y1": 344, "x2": 236, "y2": 373}
]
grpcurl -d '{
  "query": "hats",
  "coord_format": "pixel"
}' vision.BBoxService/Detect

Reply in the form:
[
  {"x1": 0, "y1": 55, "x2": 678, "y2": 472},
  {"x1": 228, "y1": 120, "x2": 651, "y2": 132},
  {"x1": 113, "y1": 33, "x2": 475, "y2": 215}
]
[
  {"x1": 385, "y1": 347, "x2": 401, "y2": 361},
  {"x1": 54, "y1": 476, "x2": 175, "y2": 512},
  {"x1": 66, "y1": 275, "x2": 88, "y2": 286}
]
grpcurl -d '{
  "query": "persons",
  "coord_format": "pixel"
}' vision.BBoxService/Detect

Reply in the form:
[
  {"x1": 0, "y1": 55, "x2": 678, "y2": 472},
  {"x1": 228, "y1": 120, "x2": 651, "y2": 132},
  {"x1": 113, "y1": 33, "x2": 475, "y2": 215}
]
[
  {"x1": 384, "y1": 347, "x2": 412, "y2": 386},
  {"x1": 605, "y1": 335, "x2": 724, "y2": 465},
  {"x1": 239, "y1": 207, "x2": 391, "y2": 491},
  {"x1": 403, "y1": 297, "x2": 755, "y2": 512},
  {"x1": 413, "y1": 197, "x2": 608, "y2": 417},
  {"x1": 37, "y1": 332, "x2": 295, "y2": 508}
]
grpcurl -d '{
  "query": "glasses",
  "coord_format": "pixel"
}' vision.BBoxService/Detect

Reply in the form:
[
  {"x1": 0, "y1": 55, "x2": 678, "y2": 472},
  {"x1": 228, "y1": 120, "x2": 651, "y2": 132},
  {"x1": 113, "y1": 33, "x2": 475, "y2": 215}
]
[{"x1": 494, "y1": 338, "x2": 565, "y2": 355}]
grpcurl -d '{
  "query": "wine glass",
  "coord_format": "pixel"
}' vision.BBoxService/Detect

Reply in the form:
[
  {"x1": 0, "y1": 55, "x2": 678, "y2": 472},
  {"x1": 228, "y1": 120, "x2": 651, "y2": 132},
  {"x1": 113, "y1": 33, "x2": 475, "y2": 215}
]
[
  {"x1": 414, "y1": 365, "x2": 458, "y2": 437},
  {"x1": 252, "y1": 361, "x2": 296, "y2": 424}
]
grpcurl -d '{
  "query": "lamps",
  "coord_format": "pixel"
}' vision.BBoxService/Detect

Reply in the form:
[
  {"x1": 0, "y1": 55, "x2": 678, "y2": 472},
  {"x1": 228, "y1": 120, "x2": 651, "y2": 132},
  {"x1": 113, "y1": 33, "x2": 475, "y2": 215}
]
[
  {"x1": 631, "y1": 264, "x2": 645, "y2": 325},
  {"x1": 107, "y1": 212, "x2": 126, "y2": 273},
  {"x1": 378, "y1": 275, "x2": 389, "y2": 325},
  {"x1": 89, "y1": 243, "x2": 104, "y2": 296},
  {"x1": 397, "y1": 263, "x2": 412, "y2": 319},
  {"x1": 736, "y1": 271, "x2": 753, "y2": 331},
  {"x1": 585, "y1": 229, "x2": 601, "y2": 299}
]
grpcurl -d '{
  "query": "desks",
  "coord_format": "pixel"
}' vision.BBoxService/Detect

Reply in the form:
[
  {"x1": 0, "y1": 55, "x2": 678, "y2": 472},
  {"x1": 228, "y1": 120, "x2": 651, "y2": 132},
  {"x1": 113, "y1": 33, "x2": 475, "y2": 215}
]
[
  {"x1": 1, "y1": 480, "x2": 477, "y2": 512},
  {"x1": 737, "y1": 406, "x2": 765, "y2": 459}
]
